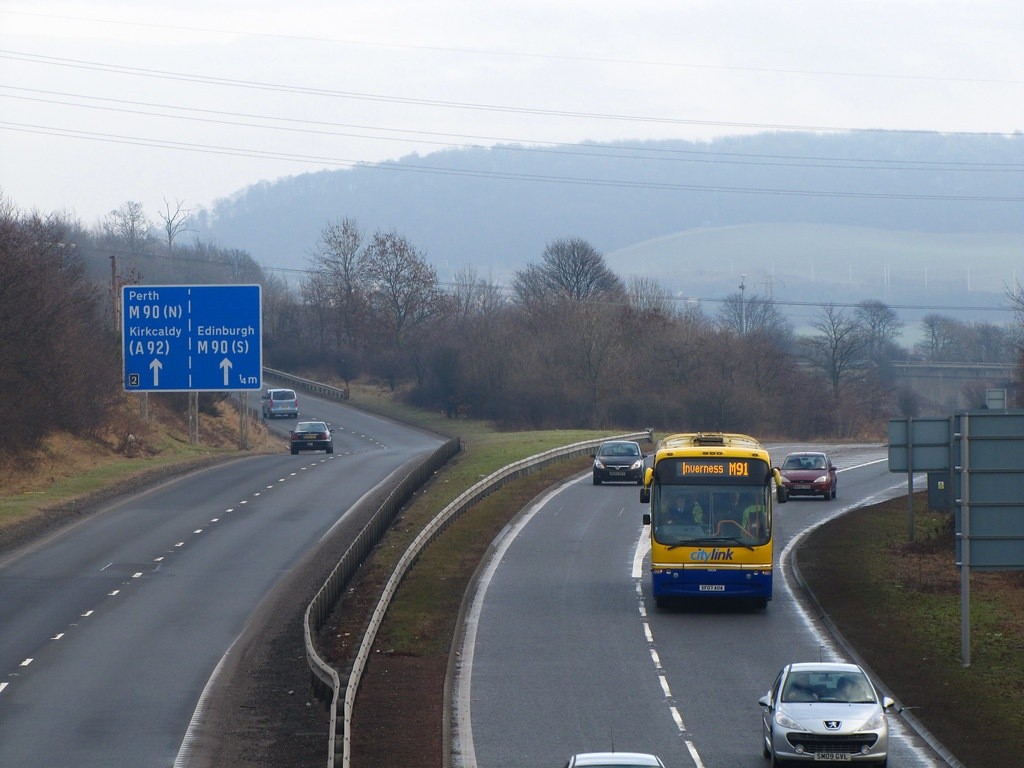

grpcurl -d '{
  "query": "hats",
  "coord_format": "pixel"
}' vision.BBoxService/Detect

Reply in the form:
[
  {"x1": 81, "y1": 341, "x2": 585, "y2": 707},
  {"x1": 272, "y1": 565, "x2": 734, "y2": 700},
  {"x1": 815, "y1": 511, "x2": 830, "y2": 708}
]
[{"x1": 843, "y1": 674, "x2": 866, "y2": 691}]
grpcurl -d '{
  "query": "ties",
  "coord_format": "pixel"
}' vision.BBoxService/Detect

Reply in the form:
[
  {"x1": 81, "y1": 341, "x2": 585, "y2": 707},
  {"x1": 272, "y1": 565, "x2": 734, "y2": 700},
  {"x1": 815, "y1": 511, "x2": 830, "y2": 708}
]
[{"x1": 679, "y1": 507, "x2": 682, "y2": 513}]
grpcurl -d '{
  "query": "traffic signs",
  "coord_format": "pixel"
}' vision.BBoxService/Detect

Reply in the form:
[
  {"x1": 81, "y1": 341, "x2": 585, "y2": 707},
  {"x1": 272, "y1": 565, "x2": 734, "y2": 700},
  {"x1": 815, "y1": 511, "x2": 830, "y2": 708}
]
[{"x1": 121, "y1": 283, "x2": 263, "y2": 392}]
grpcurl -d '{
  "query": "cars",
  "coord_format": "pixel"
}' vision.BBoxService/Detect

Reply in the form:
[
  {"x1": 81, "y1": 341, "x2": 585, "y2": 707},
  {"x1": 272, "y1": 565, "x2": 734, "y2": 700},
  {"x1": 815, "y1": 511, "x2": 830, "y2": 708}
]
[
  {"x1": 261, "y1": 388, "x2": 298, "y2": 417},
  {"x1": 758, "y1": 662, "x2": 896, "y2": 768},
  {"x1": 565, "y1": 750, "x2": 665, "y2": 768},
  {"x1": 289, "y1": 421, "x2": 335, "y2": 455},
  {"x1": 590, "y1": 441, "x2": 648, "y2": 485},
  {"x1": 776, "y1": 451, "x2": 838, "y2": 503}
]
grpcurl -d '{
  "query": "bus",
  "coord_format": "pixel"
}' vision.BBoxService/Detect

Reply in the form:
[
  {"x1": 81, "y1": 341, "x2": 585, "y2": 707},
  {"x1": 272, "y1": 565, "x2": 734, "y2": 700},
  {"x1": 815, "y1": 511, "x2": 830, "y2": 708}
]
[{"x1": 639, "y1": 433, "x2": 785, "y2": 611}]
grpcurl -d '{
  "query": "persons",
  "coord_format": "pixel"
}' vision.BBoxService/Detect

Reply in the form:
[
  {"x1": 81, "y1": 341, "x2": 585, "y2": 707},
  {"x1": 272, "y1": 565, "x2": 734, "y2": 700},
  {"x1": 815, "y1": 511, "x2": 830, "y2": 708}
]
[
  {"x1": 789, "y1": 674, "x2": 822, "y2": 700},
  {"x1": 624, "y1": 447, "x2": 635, "y2": 455},
  {"x1": 661, "y1": 490, "x2": 702, "y2": 526},
  {"x1": 739, "y1": 500, "x2": 769, "y2": 538},
  {"x1": 837, "y1": 678, "x2": 866, "y2": 700},
  {"x1": 791, "y1": 458, "x2": 802, "y2": 467}
]
